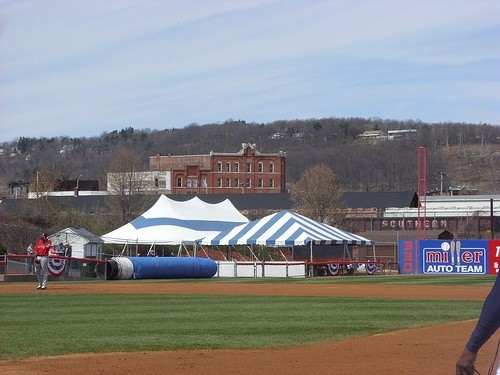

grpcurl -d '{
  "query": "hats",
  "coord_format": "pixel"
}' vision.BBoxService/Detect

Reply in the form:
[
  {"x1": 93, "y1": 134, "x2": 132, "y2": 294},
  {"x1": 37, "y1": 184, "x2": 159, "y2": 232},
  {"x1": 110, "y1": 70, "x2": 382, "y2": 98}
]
[{"x1": 40, "y1": 232, "x2": 47, "y2": 238}]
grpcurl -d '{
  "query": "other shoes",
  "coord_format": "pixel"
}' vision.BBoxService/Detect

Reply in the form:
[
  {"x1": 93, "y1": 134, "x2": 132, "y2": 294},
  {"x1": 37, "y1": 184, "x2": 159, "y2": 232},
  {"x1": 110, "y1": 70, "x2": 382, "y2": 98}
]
[
  {"x1": 41, "y1": 286, "x2": 47, "y2": 289},
  {"x1": 37, "y1": 285, "x2": 41, "y2": 289}
]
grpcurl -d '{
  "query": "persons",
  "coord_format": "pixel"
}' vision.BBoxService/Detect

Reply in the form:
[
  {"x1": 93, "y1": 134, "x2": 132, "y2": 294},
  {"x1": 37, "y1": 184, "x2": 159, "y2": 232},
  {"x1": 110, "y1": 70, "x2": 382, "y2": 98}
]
[
  {"x1": 34, "y1": 233, "x2": 55, "y2": 289},
  {"x1": 26, "y1": 241, "x2": 36, "y2": 274},
  {"x1": 56, "y1": 242, "x2": 66, "y2": 256},
  {"x1": 454, "y1": 270, "x2": 500, "y2": 375}
]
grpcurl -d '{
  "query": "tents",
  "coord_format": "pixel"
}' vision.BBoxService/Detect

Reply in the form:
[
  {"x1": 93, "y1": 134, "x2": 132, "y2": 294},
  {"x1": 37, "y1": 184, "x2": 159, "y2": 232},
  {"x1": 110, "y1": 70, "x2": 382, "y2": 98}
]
[
  {"x1": 100, "y1": 194, "x2": 254, "y2": 246},
  {"x1": 195, "y1": 209, "x2": 377, "y2": 262}
]
